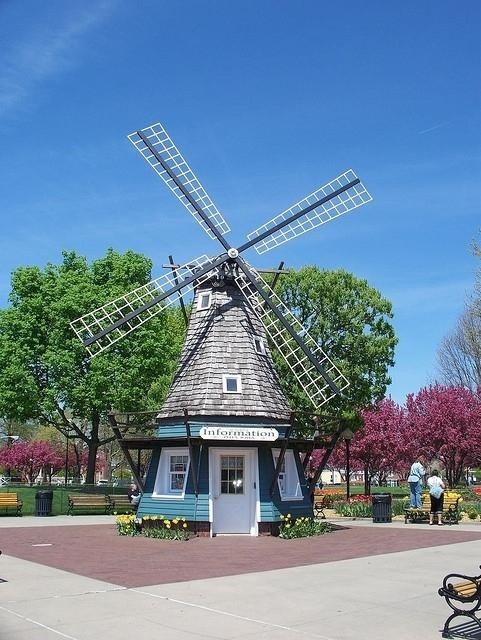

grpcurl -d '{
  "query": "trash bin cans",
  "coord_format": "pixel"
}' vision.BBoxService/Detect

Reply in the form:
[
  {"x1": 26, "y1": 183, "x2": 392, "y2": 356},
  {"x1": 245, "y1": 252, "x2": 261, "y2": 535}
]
[
  {"x1": 372, "y1": 495, "x2": 392, "y2": 523},
  {"x1": 35, "y1": 489, "x2": 53, "y2": 516}
]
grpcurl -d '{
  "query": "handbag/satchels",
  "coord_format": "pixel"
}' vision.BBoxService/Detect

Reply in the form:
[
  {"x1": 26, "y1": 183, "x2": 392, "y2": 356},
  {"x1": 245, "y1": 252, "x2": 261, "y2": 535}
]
[{"x1": 430, "y1": 484, "x2": 443, "y2": 498}]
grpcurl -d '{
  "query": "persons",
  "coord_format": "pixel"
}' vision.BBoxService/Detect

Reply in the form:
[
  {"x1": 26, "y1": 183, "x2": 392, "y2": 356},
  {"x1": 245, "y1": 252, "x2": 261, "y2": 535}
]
[
  {"x1": 127, "y1": 483, "x2": 141, "y2": 503},
  {"x1": 426, "y1": 469, "x2": 446, "y2": 526},
  {"x1": 408, "y1": 454, "x2": 427, "y2": 509}
]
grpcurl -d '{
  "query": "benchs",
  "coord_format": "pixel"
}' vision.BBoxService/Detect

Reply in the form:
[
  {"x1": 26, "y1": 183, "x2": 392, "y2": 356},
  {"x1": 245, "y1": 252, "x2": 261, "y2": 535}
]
[
  {"x1": 0, "y1": 492, "x2": 23, "y2": 517},
  {"x1": 314, "y1": 494, "x2": 331, "y2": 519},
  {"x1": 67, "y1": 494, "x2": 113, "y2": 515},
  {"x1": 108, "y1": 495, "x2": 135, "y2": 515},
  {"x1": 438, "y1": 565, "x2": 481, "y2": 640},
  {"x1": 402, "y1": 497, "x2": 460, "y2": 525}
]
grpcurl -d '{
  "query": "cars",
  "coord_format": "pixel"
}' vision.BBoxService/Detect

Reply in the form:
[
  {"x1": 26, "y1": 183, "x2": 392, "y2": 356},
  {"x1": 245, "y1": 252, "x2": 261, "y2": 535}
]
[
  {"x1": 96, "y1": 480, "x2": 108, "y2": 488},
  {"x1": 50, "y1": 478, "x2": 62, "y2": 486}
]
